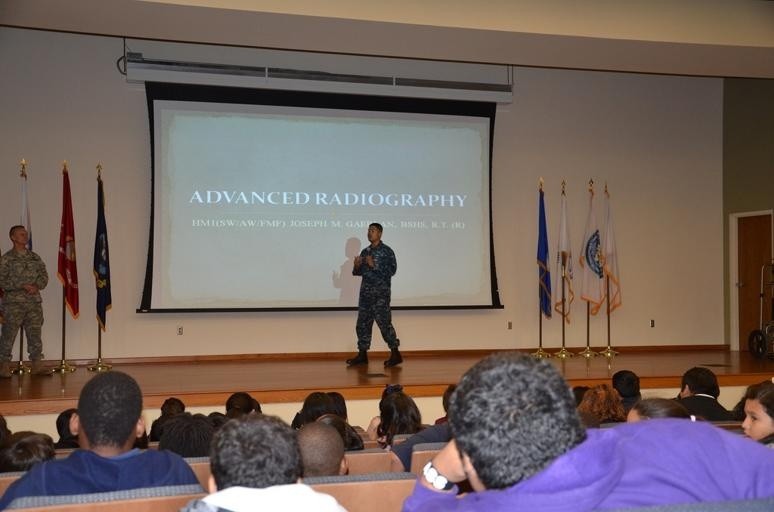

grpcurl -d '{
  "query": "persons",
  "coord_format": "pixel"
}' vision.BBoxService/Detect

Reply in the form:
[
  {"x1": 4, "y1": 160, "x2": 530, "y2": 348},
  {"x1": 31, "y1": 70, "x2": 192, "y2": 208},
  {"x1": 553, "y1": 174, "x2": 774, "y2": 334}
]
[
  {"x1": 0, "y1": 225, "x2": 53, "y2": 378},
  {"x1": 345, "y1": 222, "x2": 404, "y2": 367}
]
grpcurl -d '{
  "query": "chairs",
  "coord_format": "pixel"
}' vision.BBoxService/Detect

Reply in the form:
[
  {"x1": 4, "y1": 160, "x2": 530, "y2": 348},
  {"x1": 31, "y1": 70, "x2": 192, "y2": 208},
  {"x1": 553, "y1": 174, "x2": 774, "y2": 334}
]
[{"x1": 0, "y1": 411, "x2": 773, "y2": 510}]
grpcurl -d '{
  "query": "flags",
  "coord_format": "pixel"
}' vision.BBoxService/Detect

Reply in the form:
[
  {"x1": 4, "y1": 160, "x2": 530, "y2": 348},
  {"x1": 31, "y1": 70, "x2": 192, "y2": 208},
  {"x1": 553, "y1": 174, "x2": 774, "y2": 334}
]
[
  {"x1": 554, "y1": 191, "x2": 575, "y2": 324},
  {"x1": 536, "y1": 190, "x2": 552, "y2": 320},
  {"x1": 20, "y1": 176, "x2": 32, "y2": 251},
  {"x1": 57, "y1": 167, "x2": 81, "y2": 321},
  {"x1": 602, "y1": 190, "x2": 622, "y2": 313},
  {"x1": 579, "y1": 192, "x2": 606, "y2": 316},
  {"x1": 92, "y1": 172, "x2": 112, "y2": 333}
]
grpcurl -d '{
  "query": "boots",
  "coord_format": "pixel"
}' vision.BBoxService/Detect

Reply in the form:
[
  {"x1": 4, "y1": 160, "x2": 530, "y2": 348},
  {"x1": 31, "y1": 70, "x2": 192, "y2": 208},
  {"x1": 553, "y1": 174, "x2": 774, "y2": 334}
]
[
  {"x1": 384, "y1": 348, "x2": 403, "y2": 366},
  {"x1": 0, "y1": 364, "x2": 11, "y2": 378},
  {"x1": 30, "y1": 360, "x2": 53, "y2": 374},
  {"x1": 346, "y1": 352, "x2": 368, "y2": 365}
]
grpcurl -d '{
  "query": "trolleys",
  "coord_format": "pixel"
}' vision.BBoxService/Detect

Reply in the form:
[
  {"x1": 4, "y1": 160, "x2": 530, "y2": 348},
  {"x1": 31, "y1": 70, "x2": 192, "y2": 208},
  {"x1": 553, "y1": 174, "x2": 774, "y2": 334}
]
[{"x1": 748, "y1": 262, "x2": 774, "y2": 358}]
